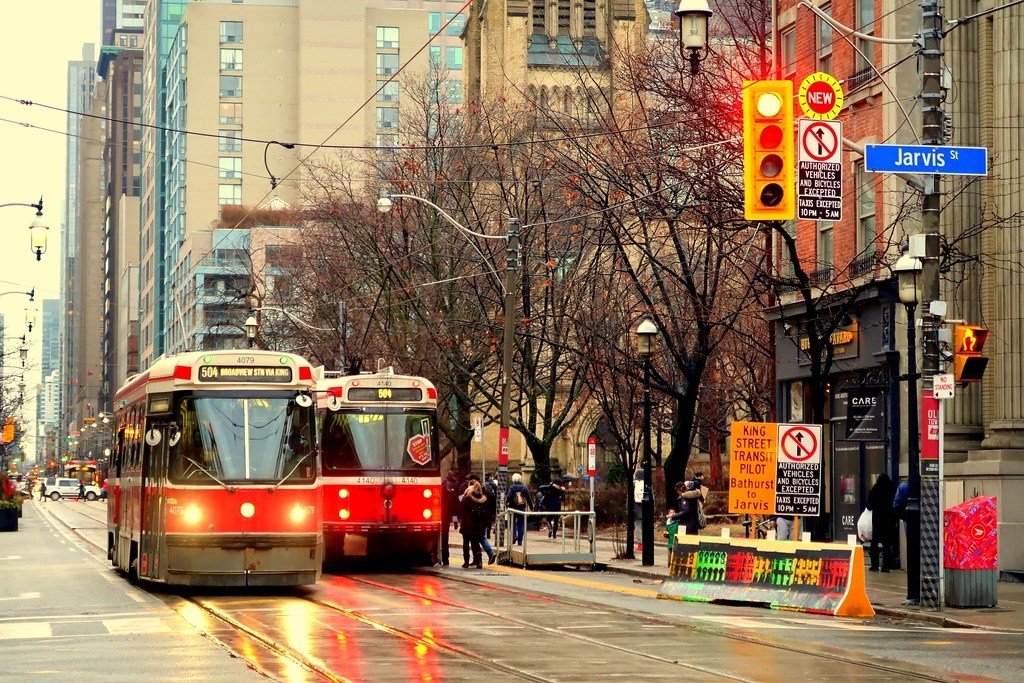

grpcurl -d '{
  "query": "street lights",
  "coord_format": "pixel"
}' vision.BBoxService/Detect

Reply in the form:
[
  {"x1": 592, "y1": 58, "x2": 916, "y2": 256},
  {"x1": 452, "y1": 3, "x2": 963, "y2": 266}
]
[
  {"x1": 244, "y1": 300, "x2": 347, "y2": 375},
  {"x1": 892, "y1": 243, "x2": 924, "y2": 606},
  {"x1": 634, "y1": 313, "x2": 662, "y2": 567},
  {"x1": 377, "y1": 192, "x2": 522, "y2": 546}
]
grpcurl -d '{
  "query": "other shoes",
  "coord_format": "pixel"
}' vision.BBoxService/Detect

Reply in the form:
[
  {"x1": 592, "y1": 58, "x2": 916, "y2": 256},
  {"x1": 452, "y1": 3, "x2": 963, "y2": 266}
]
[
  {"x1": 513, "y1": 536, "x2": 518, "y2": 543},
  {"x1": 881, "y1": 565, "x2": 889, "y2": 572},
  {"x1": 488, "y1": 553, "x2": 496, "y2": 565},
  {"x1": 869, "y1": 563, "x2": 879, "y2": 570}
]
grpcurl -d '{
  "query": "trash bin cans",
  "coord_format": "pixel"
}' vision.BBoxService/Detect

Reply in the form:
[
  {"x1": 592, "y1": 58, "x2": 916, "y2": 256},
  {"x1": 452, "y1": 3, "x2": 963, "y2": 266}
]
[{"x1": 944, "y1": 495, "x2": 998, "y2": 608}]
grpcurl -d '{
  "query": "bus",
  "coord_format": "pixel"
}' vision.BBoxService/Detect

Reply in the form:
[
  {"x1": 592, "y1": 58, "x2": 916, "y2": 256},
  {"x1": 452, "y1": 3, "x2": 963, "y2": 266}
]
[
  {"x1": 107, "y1": 349, "x2": 341, "y2": 591},
  {"x1": 315, "y1": 354, "x2": 443, "y2": 574}
]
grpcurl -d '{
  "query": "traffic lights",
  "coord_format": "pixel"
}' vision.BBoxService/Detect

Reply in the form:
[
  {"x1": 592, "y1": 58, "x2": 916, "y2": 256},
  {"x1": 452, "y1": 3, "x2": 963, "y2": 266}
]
[
  {"x1": 955, "y1": 324, "x2": 990, "y2": 382},
  {"x1": 742, "y1": 79, "x2": 796, "y2": 220}
]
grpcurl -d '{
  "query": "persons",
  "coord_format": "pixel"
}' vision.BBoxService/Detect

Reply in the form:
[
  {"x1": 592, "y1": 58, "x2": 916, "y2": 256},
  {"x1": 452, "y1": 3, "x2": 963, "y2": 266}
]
[
  {"x1": 39, "y1": 482, "x2": 47, "y2": 502},
  {"x1": 484, "y1": 473, "x2": 533, "y2": 546},
  {"x1": 663, "y1": 471, "x2": 706, "y2": 567},
  {"x1": 626, "y1": 469, "x2": 644, "y2": 551},
  {"x1": 76, "y1": 480, "x2": 86, "y2": 502},
  {"x1": 96, "y1": 484, "x2": 105, "y2": 502},
  {"x1": 534, "y1": 477, "x2": 566, "y2": 539},
  {"x1": 769, "y1": 515, "x2": 801, "y2": 540},
  {"x1": 26, "y1": 478, "x2": 34, "y2": 499},
  {"x1": 866, "y1": 473, "x2": 895, "y2": 574},
  {"x1": 441, "y1": 471, "x2": 496, "y2": 569},
  {"x1": 329, "y1": 426, "x2": 349, "y2": 467},
  {"x1": 893, "y1": 470, "x2": 921, "y2": 536}
]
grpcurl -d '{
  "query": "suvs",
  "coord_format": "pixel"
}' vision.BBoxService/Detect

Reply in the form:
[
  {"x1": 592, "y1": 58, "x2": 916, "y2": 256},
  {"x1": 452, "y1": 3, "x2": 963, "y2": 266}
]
[{"x1": 43, "y1": 477, "x2": 103, "y2": 502}]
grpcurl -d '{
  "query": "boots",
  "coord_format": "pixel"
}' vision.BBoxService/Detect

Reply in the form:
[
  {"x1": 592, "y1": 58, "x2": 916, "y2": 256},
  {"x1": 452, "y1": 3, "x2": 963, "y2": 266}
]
[
  {"x1": 462, "y1": 555, "x2": 469, "y2": 568},
  {"x1": 553, "y1": 527, "x2": 557, "y2": 538},
  {"x1": 547, "y1": 521, "x2": 552, "y2": 538},
  {"x1": 476, "y1": 553, "x2": 482, "y2": 568}
]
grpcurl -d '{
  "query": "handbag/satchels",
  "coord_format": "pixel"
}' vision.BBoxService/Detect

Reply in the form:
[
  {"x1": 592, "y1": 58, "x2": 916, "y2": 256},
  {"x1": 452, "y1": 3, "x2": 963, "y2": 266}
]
[
  {"x1": 82, "y1": 488, "x2": 86, "y2": 492},
  {"x1": 540, "y1": 493, "x2": 549, "y2": 509},
  {"x1": 858, "y1": 507, "x2": 873, "y2": 542},
  {"x1": 697, "y1": 500, "x2": 706, "y2": 529}
]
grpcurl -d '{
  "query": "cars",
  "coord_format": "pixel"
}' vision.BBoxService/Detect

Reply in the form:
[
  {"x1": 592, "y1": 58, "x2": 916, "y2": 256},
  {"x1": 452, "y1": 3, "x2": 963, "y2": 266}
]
[{"x1": 6, "y1": 470, "x2": 47, "y2": 499}]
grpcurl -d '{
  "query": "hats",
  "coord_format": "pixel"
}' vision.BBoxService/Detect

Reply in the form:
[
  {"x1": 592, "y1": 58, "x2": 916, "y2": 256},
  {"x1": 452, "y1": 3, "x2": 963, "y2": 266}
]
[{"x1": 695, "y1": 472, "x2": 704, "y2": 476}]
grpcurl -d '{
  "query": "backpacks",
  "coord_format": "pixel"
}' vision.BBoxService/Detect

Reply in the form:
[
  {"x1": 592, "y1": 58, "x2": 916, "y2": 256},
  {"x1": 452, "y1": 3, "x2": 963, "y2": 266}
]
[{"x1": 512, "y1": 485, "x2": 526, "y2": 509}]
